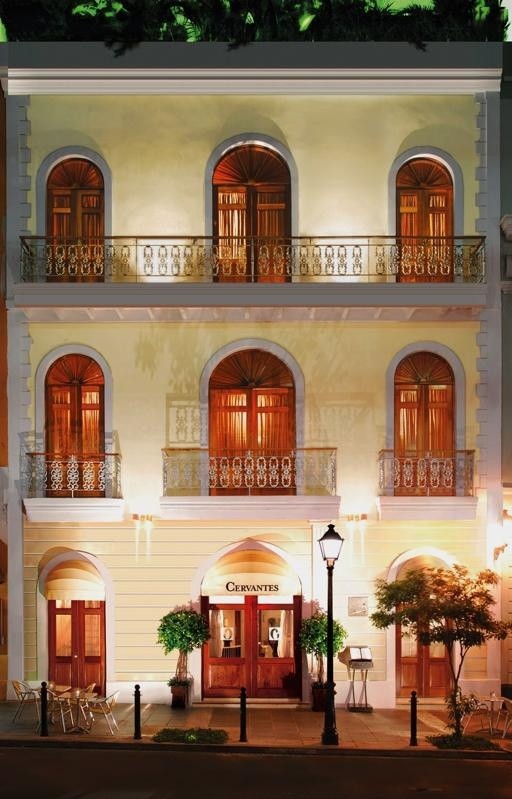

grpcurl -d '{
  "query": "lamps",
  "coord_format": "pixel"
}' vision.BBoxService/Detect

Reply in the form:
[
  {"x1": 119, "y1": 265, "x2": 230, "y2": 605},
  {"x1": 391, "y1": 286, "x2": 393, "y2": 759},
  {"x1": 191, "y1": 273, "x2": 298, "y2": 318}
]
[
  {"x1": 132, "y1": 513, "x2": 152, "y2": 523},
  {"x1": 348, "y1": 514, "x2": 369, "y2": 521}
]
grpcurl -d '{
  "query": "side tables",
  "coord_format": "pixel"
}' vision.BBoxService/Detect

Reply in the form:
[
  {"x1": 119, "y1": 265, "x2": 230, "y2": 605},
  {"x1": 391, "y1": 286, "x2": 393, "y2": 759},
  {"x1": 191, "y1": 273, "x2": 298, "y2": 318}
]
[{"x1": 480, "y1": 696, "x2": 504, "y2": 735}]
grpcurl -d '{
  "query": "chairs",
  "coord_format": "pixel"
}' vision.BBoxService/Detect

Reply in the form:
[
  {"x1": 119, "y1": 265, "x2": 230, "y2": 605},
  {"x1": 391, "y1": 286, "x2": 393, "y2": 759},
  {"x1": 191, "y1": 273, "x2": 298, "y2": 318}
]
[
  {"x1": 12, "y1": 679, "x2": 120, "y2": 736},
  {"x1": 459, "y1": 692, "x2": 494, "y2": 736},
  {"x1": 496, "y1": 696, "x2": 512, "y2": 738}
]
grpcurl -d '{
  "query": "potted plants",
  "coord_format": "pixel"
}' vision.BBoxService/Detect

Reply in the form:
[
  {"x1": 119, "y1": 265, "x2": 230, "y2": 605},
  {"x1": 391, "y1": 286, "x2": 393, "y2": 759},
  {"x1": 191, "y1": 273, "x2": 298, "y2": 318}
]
[
  {"x1": 300, "y1": 611, "x2": 349, "y2": 711},
  {"x1": 157, "y1": 611, "x2": 211, "y2": 708}
]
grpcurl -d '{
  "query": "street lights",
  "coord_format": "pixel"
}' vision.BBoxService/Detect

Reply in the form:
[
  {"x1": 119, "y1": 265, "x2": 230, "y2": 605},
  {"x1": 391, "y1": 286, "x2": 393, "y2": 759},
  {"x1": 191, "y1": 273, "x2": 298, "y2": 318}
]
[{"x1": 316, "y1": 523, "x2": 345, "y2": 745}]
